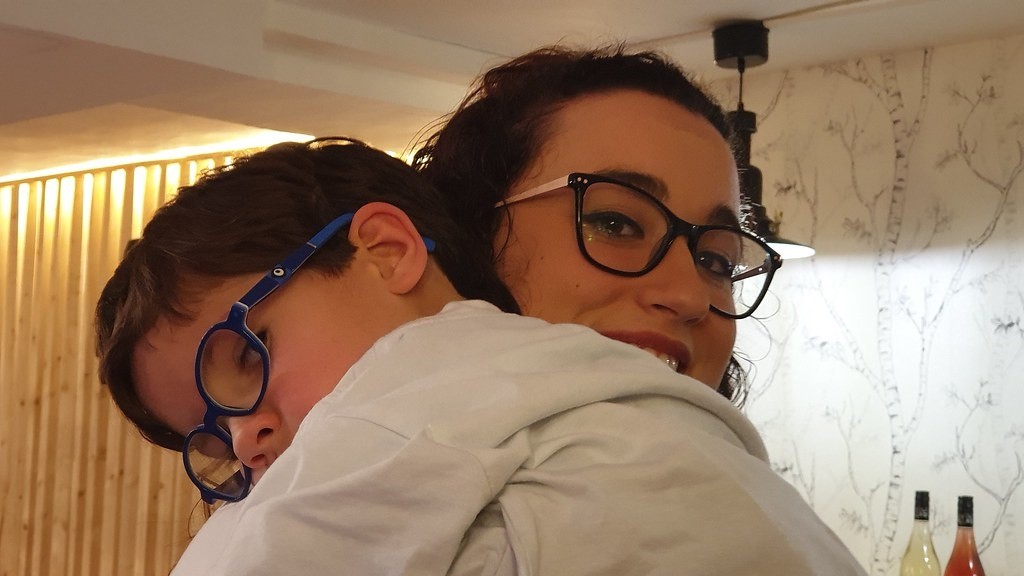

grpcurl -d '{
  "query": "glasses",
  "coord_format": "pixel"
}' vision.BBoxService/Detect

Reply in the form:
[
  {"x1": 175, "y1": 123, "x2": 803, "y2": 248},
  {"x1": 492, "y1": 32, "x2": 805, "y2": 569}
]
[
  {"x1": 182, "y1": 213, "x2": 436, "y2": 504},
  {"x1": 496, "y1": 172, "x2": 783, "y2": 319}
]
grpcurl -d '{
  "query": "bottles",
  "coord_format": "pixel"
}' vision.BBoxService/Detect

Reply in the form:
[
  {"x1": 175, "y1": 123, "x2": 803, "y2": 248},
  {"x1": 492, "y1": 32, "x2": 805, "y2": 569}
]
[
  {"x1": 943, "y1": 496, "x2": 985, "y2": 576},
  {"x1": 899, "y1": 491, "x2": 942, "y2": 576}
]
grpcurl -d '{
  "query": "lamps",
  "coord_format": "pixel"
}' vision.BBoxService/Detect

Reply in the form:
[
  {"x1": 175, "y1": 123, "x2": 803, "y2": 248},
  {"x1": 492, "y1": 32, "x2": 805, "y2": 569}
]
[{"x1": 713, "y1": 20, "x2": 816, "y2": 260}]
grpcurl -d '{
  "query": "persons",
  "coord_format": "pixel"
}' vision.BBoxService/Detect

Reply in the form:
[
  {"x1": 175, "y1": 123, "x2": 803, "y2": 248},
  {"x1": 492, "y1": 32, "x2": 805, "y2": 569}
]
[{"x1": 88, "y1": 50, "x2": 870, "y2": 574}]
